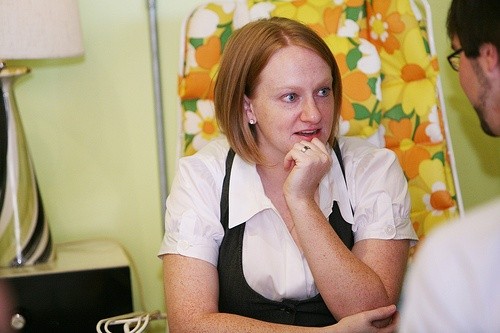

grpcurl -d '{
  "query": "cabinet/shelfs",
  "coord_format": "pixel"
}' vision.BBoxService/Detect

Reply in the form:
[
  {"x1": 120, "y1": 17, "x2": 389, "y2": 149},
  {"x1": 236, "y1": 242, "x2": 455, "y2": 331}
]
[{"x1": 1, "y1": 238, "x2": 134, "y2": 333}]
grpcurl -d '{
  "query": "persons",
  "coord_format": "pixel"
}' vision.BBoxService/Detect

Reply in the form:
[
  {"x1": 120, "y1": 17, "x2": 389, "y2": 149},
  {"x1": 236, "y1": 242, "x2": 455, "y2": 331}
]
[
  {"x1": 158, "y1": 17, "x2": 419, "y2": 333},
  {"x1": 396, "y1": 2, "x2": 500, "y2": 333}
]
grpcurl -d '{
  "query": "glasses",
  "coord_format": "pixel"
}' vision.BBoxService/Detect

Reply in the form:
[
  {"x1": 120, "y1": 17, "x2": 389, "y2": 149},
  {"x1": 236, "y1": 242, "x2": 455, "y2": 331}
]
[{"x1": 448, "y1": 47, "x2": 466, "y2": 71}]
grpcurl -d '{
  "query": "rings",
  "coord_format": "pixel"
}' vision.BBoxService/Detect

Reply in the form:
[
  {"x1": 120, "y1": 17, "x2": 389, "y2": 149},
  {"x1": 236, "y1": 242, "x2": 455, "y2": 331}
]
[{"x1": 301, "y1": 146, "x2": 310, "y2": 152}]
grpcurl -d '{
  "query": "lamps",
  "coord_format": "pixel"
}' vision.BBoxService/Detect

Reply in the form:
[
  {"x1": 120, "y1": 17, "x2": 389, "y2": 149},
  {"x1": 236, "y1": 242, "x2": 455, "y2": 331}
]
[{"x1": 0, "y1": 0, "x2": 85, "y2": 270}]
